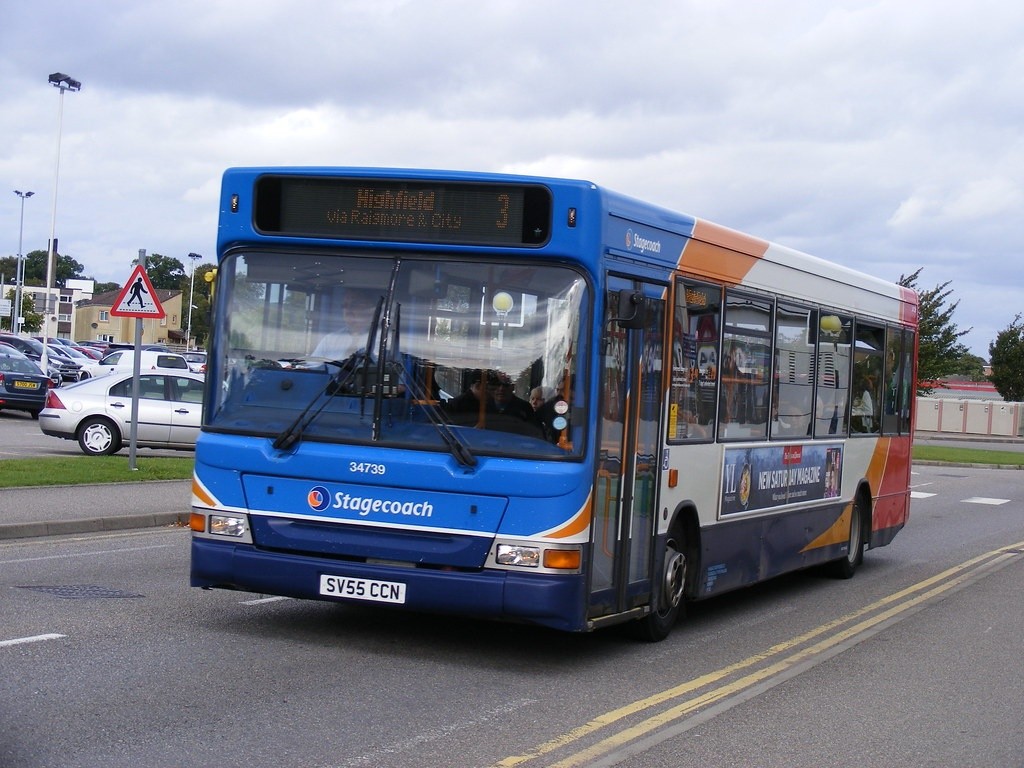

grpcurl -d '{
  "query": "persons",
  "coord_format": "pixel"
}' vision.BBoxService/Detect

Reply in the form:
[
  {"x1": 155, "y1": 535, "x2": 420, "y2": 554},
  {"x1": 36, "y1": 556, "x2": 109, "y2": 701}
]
[
  {"x1": 300, "y1": 287, "x2": 406, "y2": 397},
  {"x1": 411, "y1": 368, "x2": 574, "y2": 445},
  {"x1": 669, "y1": 316, "x2": 910, "y2": 436}
]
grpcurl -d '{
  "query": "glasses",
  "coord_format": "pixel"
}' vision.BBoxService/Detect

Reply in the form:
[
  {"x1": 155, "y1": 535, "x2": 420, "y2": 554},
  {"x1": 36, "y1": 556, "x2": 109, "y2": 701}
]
[{"x1": 342, "y1": 303, "x2": 361, "y2": 310}]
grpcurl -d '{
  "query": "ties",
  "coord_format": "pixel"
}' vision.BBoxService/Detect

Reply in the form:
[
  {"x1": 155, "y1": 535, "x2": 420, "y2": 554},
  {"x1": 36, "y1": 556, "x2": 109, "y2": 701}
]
[{"x1": 345, "y1": 337, "x2": 359, "y2": 357}]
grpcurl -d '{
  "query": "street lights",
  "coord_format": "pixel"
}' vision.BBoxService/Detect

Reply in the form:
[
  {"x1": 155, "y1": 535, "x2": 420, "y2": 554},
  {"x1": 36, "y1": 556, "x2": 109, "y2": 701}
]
[
  {"x1": 185, "y1": 252, "x2": 203, "y2": 353},
  {"x1": 39, "y1": 71, "x2": 82, "y2": 375},
  {"x1": 13, "y1": 188, "x2": 35, "y2": 333}
]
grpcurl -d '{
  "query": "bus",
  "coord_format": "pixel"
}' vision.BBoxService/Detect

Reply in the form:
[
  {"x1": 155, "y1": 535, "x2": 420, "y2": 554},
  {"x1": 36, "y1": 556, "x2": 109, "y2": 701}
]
[{"x1": 191, "y1": 165, "x2": 919, "y2": 645}]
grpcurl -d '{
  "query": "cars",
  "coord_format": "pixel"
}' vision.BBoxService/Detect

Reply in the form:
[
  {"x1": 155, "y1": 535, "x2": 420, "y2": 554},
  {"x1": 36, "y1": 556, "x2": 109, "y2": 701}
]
[{"x1": 0, "y1": 334, "x2": 229, "y2": 455}]
